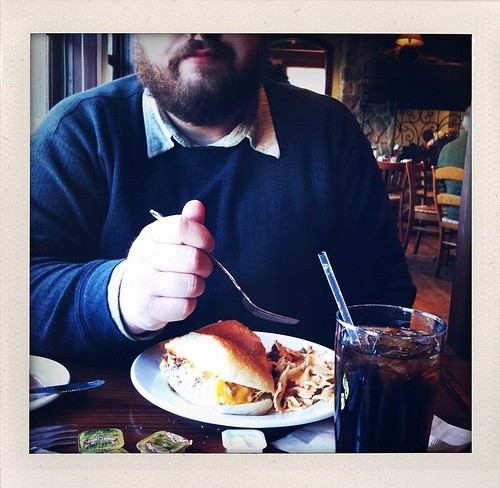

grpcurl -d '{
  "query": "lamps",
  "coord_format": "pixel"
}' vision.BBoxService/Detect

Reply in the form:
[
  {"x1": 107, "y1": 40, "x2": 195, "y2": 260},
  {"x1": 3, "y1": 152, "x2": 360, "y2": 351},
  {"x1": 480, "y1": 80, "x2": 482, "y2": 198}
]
[{"x1": 395, "y1": 35, "x2": 424, "y2": 46}]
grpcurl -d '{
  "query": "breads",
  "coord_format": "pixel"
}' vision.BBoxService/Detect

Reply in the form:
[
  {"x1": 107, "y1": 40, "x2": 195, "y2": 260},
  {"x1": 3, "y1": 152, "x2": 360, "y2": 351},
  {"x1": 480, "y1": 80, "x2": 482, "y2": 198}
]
[{"x1": 159, "y1": 320, "x2": 272, "y2": 368}]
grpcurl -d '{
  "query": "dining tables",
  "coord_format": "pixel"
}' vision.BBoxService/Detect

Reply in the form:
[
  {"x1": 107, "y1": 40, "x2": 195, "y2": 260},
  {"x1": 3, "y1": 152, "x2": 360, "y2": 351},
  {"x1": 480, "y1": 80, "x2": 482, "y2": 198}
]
[{"x1": 29, "y1": 341, "x2": 472, "y2": 452}]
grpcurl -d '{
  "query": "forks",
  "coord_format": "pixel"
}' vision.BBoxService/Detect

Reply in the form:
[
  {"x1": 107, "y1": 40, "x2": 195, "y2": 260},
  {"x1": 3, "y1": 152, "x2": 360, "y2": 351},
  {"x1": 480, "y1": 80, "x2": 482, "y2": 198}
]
[
  {"x1": 29, "y1": 423, "x2": 80, "y2": 453},
  {"x1": 149, "y1": 210, "x2": 300, "y2": 325}
]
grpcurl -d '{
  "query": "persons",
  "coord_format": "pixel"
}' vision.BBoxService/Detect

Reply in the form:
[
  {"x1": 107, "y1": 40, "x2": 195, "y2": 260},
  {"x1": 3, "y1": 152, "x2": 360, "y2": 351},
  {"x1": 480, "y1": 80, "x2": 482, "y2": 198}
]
[
  {"x1": 437, "y1": 128, "x2": 457, "y2": 151},
  {"x1": 437, "y1": 105, "x2": 473, "y2": 222},
  {"x1": 422, "y1": 129, "x2": 435, "y2": 150},
  {"x1": 30, "y1": 33, "x2": 417, "y2": 370}
]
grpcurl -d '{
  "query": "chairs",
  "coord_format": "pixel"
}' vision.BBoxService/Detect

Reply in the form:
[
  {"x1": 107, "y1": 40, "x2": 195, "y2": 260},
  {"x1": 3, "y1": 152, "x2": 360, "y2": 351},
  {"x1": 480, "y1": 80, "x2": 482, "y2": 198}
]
[
  {"x1": 377, "y1": 159, "x2": 413, "y2": 243},
  {"x1": 431, "y1": 165, "x2": 466, "y2": 277},
  {"x1": 402, "y1": 160, "x2": 447, "y2": 261}
]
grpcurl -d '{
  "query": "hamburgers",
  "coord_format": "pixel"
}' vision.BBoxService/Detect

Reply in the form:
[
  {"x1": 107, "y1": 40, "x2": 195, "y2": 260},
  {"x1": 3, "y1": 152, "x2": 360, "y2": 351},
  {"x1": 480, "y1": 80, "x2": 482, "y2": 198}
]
[{"x1": 159, "y1": 331, "x2": 276, "y2": 414}]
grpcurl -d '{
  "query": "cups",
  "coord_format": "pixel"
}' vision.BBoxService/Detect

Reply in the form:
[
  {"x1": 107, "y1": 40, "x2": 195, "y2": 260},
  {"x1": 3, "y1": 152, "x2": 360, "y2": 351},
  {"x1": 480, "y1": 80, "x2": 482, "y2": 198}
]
[{"x1": 334, "y1": 304, "x2": 448, "y2": 453}]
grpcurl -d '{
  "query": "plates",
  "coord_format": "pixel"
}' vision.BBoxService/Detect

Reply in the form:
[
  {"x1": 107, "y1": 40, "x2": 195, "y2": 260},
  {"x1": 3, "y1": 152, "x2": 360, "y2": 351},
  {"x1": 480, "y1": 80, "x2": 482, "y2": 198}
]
[
  {"x1": 29, "y1": 354, "x2": 71, "y2": 412},
  {"x1": 130, "y1": 331, "x2": 335, "y2": 428}
]
